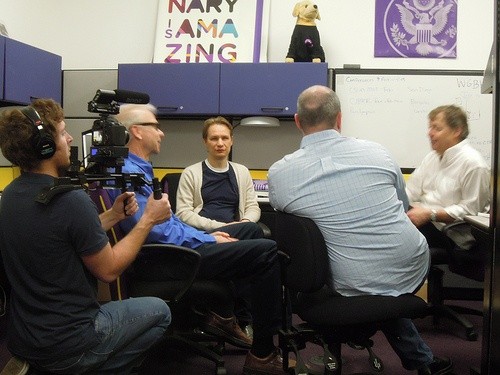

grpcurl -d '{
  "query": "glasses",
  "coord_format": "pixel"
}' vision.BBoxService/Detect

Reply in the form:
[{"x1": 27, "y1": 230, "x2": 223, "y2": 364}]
[{"x1": 127, "y1": 123, "x2": 160, "y2": 132}]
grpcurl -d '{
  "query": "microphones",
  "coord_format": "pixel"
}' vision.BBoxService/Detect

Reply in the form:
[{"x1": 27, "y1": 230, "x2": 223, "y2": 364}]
[{"x1": 94, "y1": 89, "x2": 150, "y2": 104}]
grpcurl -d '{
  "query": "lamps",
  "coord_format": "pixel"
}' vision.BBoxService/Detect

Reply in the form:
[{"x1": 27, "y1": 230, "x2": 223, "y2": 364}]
[{"x1": 233, "y1": 116, "x2": 281, "y2": 131}]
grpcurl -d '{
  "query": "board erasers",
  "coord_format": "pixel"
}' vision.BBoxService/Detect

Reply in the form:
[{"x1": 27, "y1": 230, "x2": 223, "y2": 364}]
[{"x1": 344, "y1": 64, "x2": 361, "y2": 68}]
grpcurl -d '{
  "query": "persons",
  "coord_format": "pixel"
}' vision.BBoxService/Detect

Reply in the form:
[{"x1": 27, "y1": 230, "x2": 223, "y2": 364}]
[
  {"x1": 107, "y1": 104, "x2": 311, "y2": 375},
  {"x1": 266, "y1": 85, "x2": 454, "y2": 375},
  {"x1": 402, "y1": 104, "x2": 491, "y2": 248},
  {"x1": 174, "y1": 118, "x2": 262, "y2": 344},
  {"x1": 0, "y1": 97, "x2": 172, "y2": 375}
]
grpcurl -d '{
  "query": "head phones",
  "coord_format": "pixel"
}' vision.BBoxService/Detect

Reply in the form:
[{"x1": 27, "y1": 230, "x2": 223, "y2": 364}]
[{"x1": 20, "y1": 106, "x2": 56, "y2": 159}]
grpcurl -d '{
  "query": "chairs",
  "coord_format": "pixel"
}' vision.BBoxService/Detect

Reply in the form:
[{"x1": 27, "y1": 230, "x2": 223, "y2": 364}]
[{"x1": 0, "y1": 173, "x2": 492, "y2": 375}]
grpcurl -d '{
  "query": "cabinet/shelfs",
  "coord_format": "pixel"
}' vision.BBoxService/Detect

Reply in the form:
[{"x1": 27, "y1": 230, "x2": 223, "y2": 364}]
[
  {"x1": 118, "y1": 63, "x2": 330, "y2": 121},
  {"x1": 0, "y1": 34, "x2": 62, "y2": 107}
]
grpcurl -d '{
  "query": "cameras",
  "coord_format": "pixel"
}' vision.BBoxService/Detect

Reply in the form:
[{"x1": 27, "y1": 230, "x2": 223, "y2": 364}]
[{"x1": 92, "y1": 118, "x2": 129, "y2": 146}]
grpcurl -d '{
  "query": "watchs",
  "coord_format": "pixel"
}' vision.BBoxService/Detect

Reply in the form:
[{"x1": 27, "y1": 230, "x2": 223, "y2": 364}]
[{"x1": 431, "y1": 209, "x2": 438, "y2": 221}]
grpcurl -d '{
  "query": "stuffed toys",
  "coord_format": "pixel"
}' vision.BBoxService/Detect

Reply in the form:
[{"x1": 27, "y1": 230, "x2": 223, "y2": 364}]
[{"x1": 286, "y1": 0, "x2": 326, "y2": 63}]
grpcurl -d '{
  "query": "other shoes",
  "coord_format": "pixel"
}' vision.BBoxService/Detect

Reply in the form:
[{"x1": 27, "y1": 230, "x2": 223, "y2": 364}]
[
  {"x1": 0, "y1": 356, "x2": 33, "y2": 375},
  {"x1": 201, "y1": 310, "x2": 253, "y2": 347},
  {"x1": 243, "y1": 349, "x2": 309, "y2": 375},
  {"x1": 418, "y1": 356, "x2": 457, "y2": 375}
]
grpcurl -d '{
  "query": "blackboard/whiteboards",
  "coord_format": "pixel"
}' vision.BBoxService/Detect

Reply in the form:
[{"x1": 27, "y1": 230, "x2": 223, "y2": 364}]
[{"x1": 328, "y1": 68, "x2": 496, "y2": 173}]
